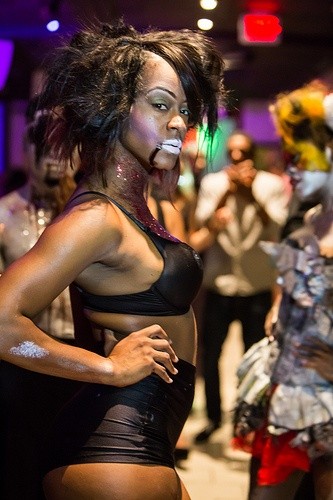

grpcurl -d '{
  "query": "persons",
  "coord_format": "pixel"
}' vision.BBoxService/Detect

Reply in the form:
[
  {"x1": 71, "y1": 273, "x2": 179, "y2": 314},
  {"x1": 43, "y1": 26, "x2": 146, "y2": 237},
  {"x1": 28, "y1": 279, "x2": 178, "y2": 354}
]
[
  {"x1": 0, "y1": 81, "x2": 333, "y2": 500},
  {"x1": 0, "y1": 19, "x2": 225, "y2": 500}
]
